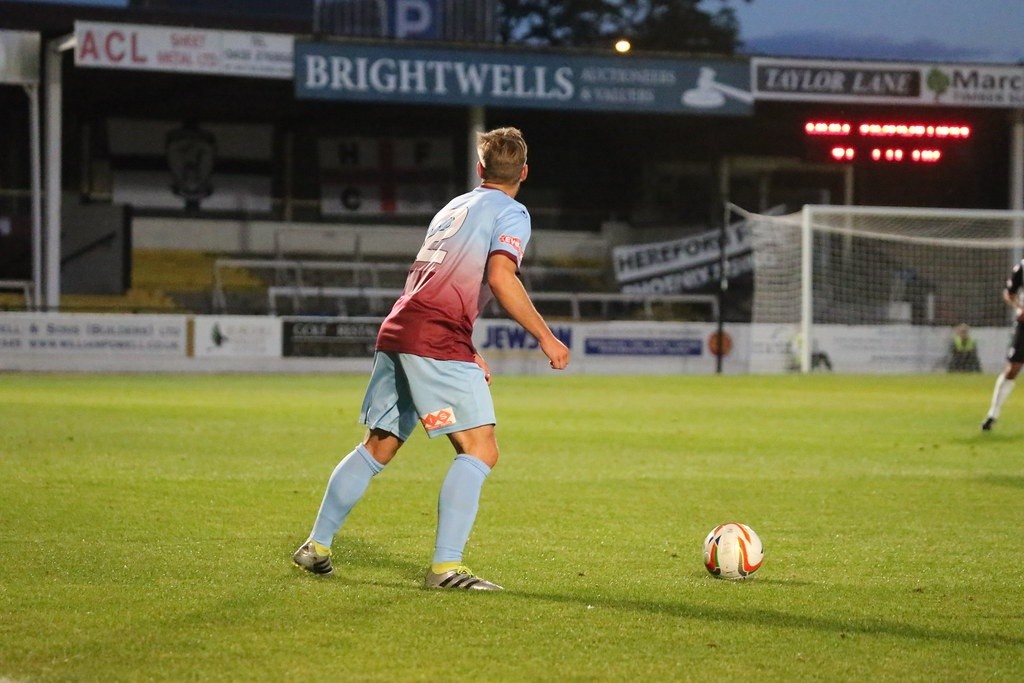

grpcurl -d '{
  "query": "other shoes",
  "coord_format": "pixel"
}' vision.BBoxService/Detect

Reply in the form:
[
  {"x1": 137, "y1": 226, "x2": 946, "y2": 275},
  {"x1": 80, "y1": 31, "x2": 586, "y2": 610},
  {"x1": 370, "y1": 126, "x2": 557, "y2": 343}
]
[{"x1": 983, "y1": 414, "x2": 996, "y2": 432}]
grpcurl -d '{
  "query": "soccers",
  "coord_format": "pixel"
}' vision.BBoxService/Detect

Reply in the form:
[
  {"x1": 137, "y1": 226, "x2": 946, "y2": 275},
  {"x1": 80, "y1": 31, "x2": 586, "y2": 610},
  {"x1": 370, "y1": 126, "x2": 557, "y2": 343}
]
[{"x1": 700, "y1": 521, "x2": 764, "y2": 579}]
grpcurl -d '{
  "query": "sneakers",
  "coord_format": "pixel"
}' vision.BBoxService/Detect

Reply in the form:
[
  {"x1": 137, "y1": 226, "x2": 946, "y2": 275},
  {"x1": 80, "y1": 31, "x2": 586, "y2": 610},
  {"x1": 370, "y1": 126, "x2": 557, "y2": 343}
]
[
  {"x1": 292, "y1": 541, "x2": 335, "y2": 576},
  {"x1": 424, "y1": 566, "x2": 505, "y2": 593}
]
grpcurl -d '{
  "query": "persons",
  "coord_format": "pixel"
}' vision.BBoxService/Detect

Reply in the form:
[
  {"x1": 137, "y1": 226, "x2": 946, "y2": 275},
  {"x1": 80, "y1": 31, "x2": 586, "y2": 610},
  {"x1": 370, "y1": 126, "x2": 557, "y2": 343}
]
[
  {"x1": 786, "y1": 327, "x2": 833, "y2": 376},
  {"x1": 946, "y1": 323, "x2": 983, "y2": 374},
  {"x1": 294, "y1": 123, "x2": 569, "y2": 592},
  {"x1": 981, "y1": 258, "x2": 1024, "y2": 431}
]
